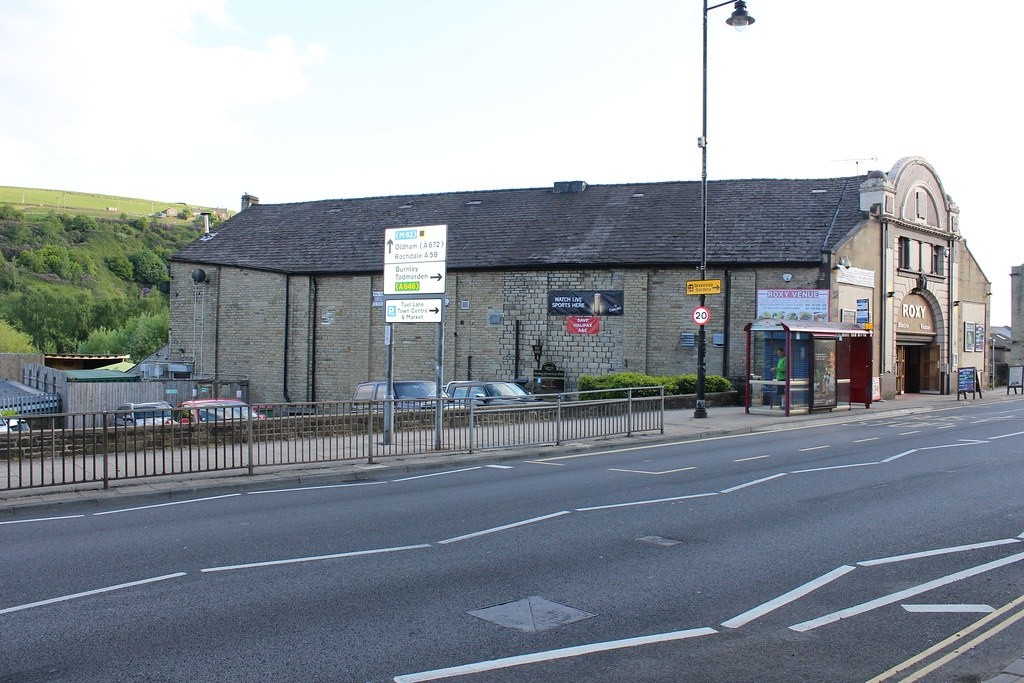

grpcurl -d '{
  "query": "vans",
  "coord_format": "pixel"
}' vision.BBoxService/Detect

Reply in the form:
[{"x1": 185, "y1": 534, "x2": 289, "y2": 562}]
[{"x1": 351, "y1": 380, "x2": 552, "y2": 413}]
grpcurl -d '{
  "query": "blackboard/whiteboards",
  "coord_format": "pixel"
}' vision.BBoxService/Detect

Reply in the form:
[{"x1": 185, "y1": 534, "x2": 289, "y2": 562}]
[{"x1": 957, "y1": 366, "x2": 982, "y2": 394}]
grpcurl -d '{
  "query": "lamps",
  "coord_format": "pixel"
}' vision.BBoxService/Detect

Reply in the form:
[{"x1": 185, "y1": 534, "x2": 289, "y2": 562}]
[
  {"x1": 192, "y1": 270, "x2": 210, "y2": 285},
  {"x1": 887, "y1": 292, "x2": 896, "y2": 297},
  {"x1": 944, "y1": 247, "x2": 950, "y2": 257},
  {"x1": 954, "y1": 301, "x2": 961, "y2": 306}
]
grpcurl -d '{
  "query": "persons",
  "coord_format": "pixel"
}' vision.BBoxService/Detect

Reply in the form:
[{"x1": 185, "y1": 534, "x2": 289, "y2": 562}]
[
  {"x1": 828, "y1": 350, "x2": 836, "y2": 393},
  {"x1": 770, "y1": 347, "x2": 786, "y2": 410}
]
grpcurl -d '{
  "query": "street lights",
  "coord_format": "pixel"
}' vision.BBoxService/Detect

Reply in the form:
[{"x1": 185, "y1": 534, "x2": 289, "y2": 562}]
[{"x1": 686, "y1": 0, "x2": 756, "y2": 419}]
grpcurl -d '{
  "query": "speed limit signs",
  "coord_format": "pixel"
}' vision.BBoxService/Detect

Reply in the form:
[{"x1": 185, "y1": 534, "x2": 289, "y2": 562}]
[{"x1": 692, "y1": 306, "x2": 710, "y2": 325}]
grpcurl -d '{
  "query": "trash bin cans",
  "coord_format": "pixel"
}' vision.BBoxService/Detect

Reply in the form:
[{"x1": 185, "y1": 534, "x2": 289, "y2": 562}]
[{"x1": 515, "y1": 378, "x2": 528, "y2": 389}]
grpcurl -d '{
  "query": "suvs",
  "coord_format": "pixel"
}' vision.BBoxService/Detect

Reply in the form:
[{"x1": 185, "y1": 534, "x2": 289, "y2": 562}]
[
  {"x1": 110, "y1": 401, "x2": 174, "y2": 426},
  {"x1": 174, "y1": 398, "x2": 266, "y2": 423}
]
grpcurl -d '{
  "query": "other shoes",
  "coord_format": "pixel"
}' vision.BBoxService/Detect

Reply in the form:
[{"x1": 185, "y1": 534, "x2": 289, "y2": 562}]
[{"x1": 779, "y1": 405, "x2": 785, "y2": 409}]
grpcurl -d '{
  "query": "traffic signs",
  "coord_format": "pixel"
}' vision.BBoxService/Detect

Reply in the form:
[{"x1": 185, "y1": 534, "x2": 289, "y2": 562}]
[
  {"x1": 383, "y1": 224, "x2": 447, "y2": 295},
  {"x1": 385, "y1": 299, "x2": 441, "y2": 323}
]
